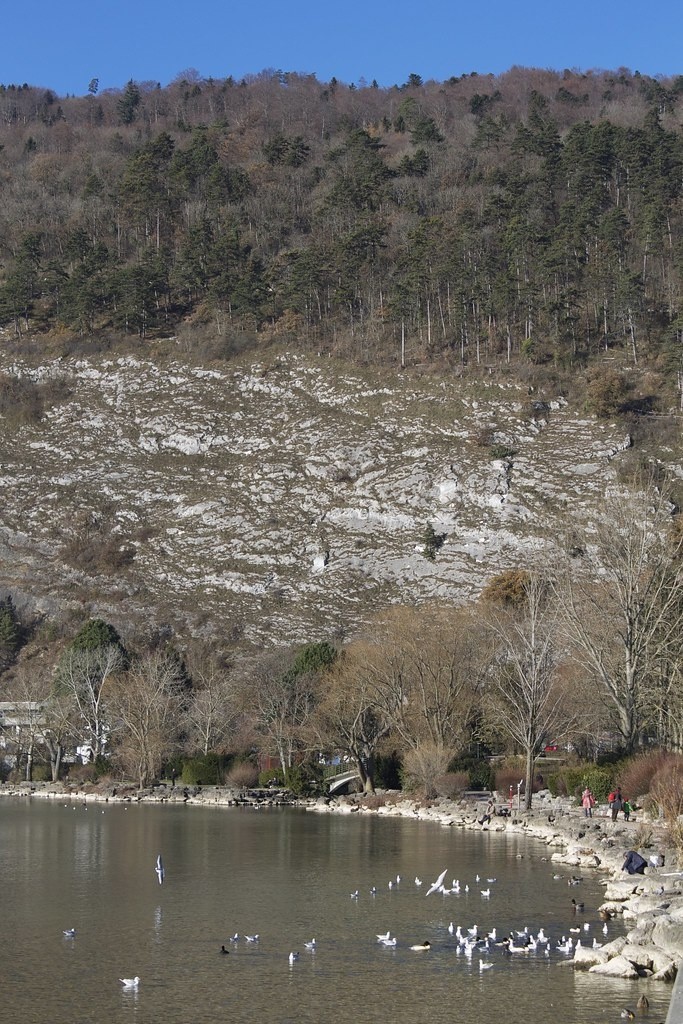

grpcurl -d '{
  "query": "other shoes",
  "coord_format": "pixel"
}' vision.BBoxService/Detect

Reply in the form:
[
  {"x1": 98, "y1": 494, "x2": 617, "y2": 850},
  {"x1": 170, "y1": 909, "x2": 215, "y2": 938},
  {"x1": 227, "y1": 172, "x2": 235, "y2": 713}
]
[
  {"x1": 478, "y1": 820, "x2": 483, "y2": 825},
  {"x1": 613, "y1": 819, "x2": 618, "y2": 822}
]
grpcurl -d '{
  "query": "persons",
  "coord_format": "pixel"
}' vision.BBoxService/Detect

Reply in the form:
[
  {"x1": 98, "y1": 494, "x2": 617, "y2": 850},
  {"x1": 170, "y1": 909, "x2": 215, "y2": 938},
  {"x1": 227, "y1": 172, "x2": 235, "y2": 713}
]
[
  {"x1": 171, "y1": 768, "x2": 176, "y2": 786},
  {"x1": 610, "y1": 787, "x2": 622, "y2": 822},
  {"x1": 478, "y1": 801, "x2": 495, "y2": 825},
  {"x1": 267, "y1": 777, "x2": 277, "y2": 789},
  {"x1": 582, "y1": 786, "x2": 593, "y2": 818},
  {"x1": 621, "y1": 851, "x2": 648, "y2": 875},
  {"x1": 319, "y1": 751, "x2": 350, "y2": 765},
  {"x1": 623, "y1": 798, "x2": 633, "y2": 822}
]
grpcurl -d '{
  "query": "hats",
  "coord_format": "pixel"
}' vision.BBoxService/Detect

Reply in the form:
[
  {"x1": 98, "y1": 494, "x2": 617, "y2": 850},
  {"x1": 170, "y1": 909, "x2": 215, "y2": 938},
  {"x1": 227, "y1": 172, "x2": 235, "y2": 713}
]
[
  {"x1": 488, "y1": 801, "x2": 492, "y2": 804},
  {"x1": 624, "y1": 798, "x2": 628, "y2": 802}
]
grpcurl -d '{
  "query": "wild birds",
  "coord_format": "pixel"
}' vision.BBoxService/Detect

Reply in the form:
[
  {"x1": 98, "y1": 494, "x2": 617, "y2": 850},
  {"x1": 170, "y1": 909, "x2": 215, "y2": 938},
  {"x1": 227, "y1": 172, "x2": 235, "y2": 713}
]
[
  {"x1": 152, "y1": 853, "x2": 168, "y2": 887},
  {"x1": 350, "y1": 867, "x2": 499, "y2": 901},
  {"x1": 514, "y1": 852, "x2": 549, "y2": 862},
  {"x1": 286, "y1": 936, "x2": 320, "y2": 964},
  {"x1": 656, "y1": 901, "x2": 673, "y2": 913},
  {"x1": 649, "y1": 855, "x2": 658, "y2": 868},
  {"x1": 597, "y1": 908, "x2": 611, "y2": 922},
  {"x1": 570, "y1": 897, "x2": 585, "y2": 911},
  {"x1": 549, "y1": 872, "x2": 585, "y2": 887},
  {"x1": 375, "y1": 923, "x2": 610, "y2": 973},
  {"x1": 620, "y1": 993, "x2": 650, "y2": 1020},
  {"x1": 217, "y1": 931, "x2": 263, "y2": 956},
  {"x1": 117, "y1": 975, "x2": 142, "y2": 990},
  {"x1": 59, "y1": 925, "x2": 78, "y2": 939}
]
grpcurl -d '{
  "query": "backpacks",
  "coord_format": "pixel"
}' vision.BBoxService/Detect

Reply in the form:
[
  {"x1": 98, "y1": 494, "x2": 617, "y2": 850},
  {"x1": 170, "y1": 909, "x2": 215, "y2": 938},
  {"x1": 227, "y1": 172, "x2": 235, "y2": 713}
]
[{"x1": 609, "y1": 793, "x2": 615, "y2": 802}]
